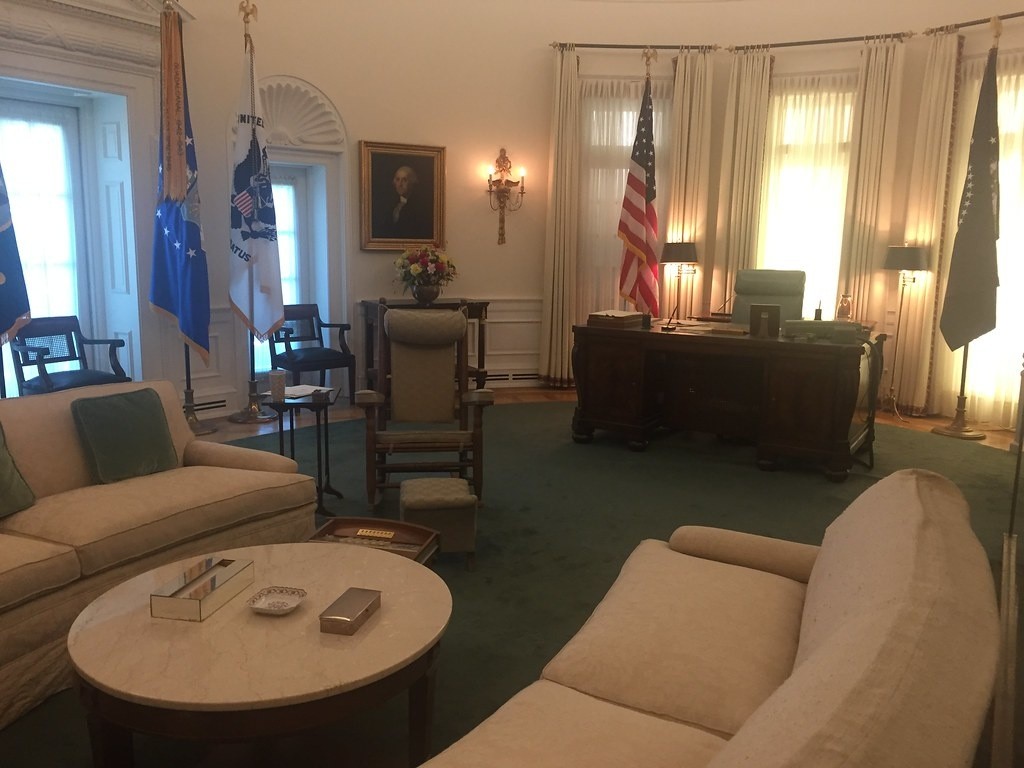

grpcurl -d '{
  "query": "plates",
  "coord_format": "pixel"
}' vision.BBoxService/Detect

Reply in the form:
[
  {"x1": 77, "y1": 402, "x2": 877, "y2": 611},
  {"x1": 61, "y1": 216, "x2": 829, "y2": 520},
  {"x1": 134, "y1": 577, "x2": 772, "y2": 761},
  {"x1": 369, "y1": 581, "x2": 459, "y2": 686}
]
[{"x1": 245, "y1": 586, "x2": 307, "y2": 614}]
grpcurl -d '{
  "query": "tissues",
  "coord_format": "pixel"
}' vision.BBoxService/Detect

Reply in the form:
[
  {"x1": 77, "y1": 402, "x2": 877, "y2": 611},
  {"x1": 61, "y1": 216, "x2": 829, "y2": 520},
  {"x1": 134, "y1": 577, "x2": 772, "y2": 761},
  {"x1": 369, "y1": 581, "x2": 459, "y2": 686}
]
[{"x1": 150, "y1": 556, "x2": 255, "y2": 622}]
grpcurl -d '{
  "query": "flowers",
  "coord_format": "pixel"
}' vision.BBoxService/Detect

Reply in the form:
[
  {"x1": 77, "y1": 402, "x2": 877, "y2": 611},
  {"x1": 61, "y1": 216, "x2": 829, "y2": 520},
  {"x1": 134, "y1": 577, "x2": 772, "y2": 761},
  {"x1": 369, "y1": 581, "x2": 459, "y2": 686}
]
[{"x1": 396, "y1": 248, "x2": 455, "y2": 285}]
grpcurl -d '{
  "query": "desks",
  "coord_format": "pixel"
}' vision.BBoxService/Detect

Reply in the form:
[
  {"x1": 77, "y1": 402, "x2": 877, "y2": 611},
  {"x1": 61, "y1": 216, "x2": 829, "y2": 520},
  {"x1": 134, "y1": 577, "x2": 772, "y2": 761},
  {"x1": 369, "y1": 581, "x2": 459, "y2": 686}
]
[{"x1": 571, "y1": 319, "x2": 887, "y2": 481}]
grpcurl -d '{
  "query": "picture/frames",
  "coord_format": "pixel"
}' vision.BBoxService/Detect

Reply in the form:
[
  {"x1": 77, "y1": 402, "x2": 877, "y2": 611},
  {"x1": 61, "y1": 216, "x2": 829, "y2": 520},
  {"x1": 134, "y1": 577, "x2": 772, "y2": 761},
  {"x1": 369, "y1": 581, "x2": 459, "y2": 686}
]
[
  {"x1": 359, "y1": 139, "x2": 445, "y2": 252},
  {"x1": 411, "y1": 282, "x2": 440, "y2": 302}
]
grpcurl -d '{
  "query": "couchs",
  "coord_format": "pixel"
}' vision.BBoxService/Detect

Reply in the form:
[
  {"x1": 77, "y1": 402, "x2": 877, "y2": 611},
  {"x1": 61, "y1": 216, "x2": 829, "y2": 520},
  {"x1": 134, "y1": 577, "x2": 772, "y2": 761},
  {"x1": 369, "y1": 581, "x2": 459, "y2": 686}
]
[
  {"x1": 0, "y1": 380, "x2": 317, "y2": 730},
  {"x1": 417, "y1": 468, "x2": 1002, "y2": 767}
]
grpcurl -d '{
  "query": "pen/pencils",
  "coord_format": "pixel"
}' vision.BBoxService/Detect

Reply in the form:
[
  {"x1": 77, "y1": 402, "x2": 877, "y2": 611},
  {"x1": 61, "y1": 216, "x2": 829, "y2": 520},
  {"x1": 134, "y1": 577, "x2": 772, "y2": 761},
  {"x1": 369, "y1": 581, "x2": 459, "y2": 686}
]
[{"x1": 667, "y1": 306, "x2": 678, "y2": 326}]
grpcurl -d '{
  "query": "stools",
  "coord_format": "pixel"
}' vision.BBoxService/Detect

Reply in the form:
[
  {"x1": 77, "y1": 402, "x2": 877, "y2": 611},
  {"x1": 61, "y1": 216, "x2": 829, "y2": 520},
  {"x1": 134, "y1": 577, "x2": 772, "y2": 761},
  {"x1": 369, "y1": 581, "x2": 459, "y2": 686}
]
[{"x1": 398, "y1": 479, "x2": 477, "y2": 555}]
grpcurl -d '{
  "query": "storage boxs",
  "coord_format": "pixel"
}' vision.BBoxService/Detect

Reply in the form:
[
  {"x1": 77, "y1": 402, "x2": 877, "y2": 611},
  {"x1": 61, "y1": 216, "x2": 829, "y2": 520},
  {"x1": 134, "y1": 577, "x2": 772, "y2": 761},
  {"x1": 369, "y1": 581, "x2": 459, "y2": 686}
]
[{"x1": 319, "y1": 586, "x2": 381, "y2": 636}]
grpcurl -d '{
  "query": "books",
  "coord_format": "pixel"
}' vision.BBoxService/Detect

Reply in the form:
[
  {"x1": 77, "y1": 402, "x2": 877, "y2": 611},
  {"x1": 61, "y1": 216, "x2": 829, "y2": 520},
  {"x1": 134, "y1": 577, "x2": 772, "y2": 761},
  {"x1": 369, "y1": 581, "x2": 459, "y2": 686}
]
[
  {"x1": 587, "y1": 310, "x2": 643, "y2": 328},
  {"x1": 261, "y1": 385, "x2": 335, "y2": 398}
]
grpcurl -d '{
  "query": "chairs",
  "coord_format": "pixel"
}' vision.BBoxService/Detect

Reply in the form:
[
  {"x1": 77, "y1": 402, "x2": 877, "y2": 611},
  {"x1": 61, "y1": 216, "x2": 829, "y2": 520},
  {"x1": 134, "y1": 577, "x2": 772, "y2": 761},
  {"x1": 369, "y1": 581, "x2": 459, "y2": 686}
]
[
  {"x1": 269, "y1": 303, "x2": 355, "y2": 414},
  {"x1": 10, "y1": 315, "x2": 131, "y2": 395},
  {"x1": 732, "y1": 270, "x2": 806, "y2": 319},
  {"x1": 356, "y1": 302, "x2": 494, "y2": 506}
]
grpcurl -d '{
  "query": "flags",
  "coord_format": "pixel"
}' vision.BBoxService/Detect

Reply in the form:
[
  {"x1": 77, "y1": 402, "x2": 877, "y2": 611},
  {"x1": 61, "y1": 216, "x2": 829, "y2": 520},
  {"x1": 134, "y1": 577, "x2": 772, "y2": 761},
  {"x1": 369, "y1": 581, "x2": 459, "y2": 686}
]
[
  {"x1": 229, "y1": 33, "x2": 284, "y2": 344},
  {"x1": 618, "y1": 73, "x2": 659, "y2": 318},
  {"x1": 941, "y1": 45, "x2": 1001, "y2": 352},
  {"x1": 0, "y1": 163, "x2": 31, "y2": 348},
  {"x1": 148, "y1": 11, "x2": 210, "y2": 368}
]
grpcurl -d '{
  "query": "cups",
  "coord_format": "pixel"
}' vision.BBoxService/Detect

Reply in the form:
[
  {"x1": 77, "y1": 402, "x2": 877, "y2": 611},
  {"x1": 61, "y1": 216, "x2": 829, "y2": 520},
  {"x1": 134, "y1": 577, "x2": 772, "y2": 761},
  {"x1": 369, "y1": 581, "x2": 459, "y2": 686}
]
[
  {"x1": 268, "y1": 369, "x2": 285, "y2": 401},
  {"x1": 642, "y1": 314, "x2": 651, "y2": 329}
]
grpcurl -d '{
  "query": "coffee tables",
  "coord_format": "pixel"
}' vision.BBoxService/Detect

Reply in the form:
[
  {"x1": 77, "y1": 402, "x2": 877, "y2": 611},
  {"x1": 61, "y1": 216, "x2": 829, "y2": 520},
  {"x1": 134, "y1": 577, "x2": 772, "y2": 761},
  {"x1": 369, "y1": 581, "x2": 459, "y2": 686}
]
[
  {"x1": 263, "y1": 394, "x2": 343, "y2": 516},
  {"x1": 67, "y1": 541, "x2": 452, "y2": 768}
]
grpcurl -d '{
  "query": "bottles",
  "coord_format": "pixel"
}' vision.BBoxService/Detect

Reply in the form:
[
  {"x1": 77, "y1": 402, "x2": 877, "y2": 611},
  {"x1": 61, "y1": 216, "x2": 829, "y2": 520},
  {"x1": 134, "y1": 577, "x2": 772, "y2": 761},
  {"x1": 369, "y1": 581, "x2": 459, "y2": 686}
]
[
  {"x1": 837, "y1": 294, "x2": 851, "y2": 320},
  {"x1": 814, "y1": 309, "x2": 822, "y2": 321}
]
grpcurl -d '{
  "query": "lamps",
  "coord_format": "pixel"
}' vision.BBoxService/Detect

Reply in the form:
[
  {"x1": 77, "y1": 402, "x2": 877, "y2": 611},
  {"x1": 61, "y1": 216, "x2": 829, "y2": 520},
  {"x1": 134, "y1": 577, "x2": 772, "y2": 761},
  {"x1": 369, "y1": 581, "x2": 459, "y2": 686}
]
[
  {"x1": 662, "y1": 243, "x2": 699, "y2": 319},
  {"x1": 881, "y1": 246, "x2": 928, "y2": 422},
  {"x1": 487, "y1": 149, "x2": 524, "y2": 246}
]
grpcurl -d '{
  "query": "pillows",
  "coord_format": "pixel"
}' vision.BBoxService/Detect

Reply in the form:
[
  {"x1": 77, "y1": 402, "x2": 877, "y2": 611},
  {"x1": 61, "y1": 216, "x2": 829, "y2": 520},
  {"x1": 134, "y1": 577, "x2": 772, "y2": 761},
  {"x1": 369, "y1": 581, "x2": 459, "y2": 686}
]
[
  {"x1": 72, "y1": 389, "x2": 178, "y2": 482},
  {"x1": 0, "y1": 423, "x2": 35, "y2": 520}
]
